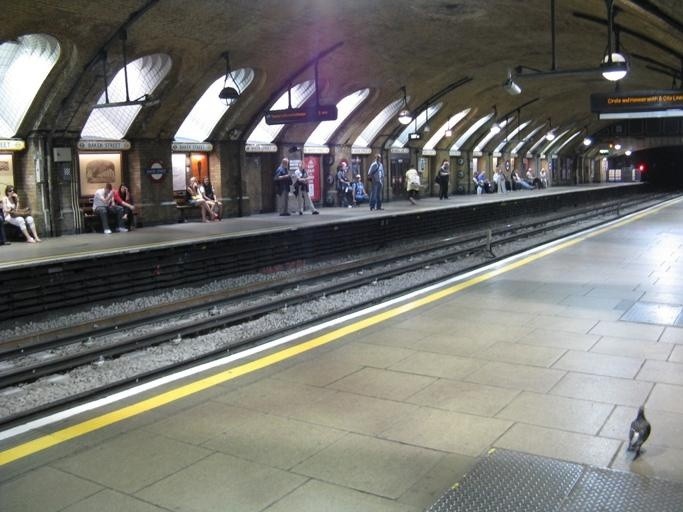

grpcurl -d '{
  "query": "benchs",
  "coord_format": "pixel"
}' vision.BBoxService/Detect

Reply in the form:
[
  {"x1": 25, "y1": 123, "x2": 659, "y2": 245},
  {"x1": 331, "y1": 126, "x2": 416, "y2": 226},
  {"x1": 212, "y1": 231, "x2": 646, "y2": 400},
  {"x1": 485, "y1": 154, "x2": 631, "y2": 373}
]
[
  {"x1": 0, "y1": 215, "x2": 23, "y2": 244},
  {"x1": 79, "y1": 198, "x2": 140, "y2": 232},
  {"x1": 172, "y1": 191, "x2": 224, "y2": 222}
]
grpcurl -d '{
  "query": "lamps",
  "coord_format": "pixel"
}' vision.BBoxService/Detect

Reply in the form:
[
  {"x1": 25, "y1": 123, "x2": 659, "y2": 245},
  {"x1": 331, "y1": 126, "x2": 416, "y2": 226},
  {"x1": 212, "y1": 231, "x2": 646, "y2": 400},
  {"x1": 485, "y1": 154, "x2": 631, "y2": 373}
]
[
  {"x1": 484, "y1": 101, "x2": 632, "y2": 156},
  {"x1": 599, "y1": 29, "x2": 626, "y2": 82},
  {"x1": 219, "y1": 55, "x2": 240, "y2": 106},
  {"x1": 444, "y1": 117, "x2": 452, "y2": 137},
  {"x1": 423, "y1": 104, "x2": 430, "y2": 131},
  {"x1": 395, "y1": 85, "x2": 412, "y2": 125}
]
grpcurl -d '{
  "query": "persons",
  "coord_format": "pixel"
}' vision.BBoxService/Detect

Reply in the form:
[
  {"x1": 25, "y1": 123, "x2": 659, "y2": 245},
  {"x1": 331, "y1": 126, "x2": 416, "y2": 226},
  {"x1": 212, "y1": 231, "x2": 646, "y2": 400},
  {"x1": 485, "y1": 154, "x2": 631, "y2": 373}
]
[
  {"x1": 350, "y1": 173, "x2": 369, "y2": 204},
  {"x1": 367, "y1": 153, "x2": 385, "y2": 211},
  {"x1": 2, "y1": 185, "x2": 41, "y2": 243},
  {"x1": 114, "y1": 185, "x2": 137, "y2": 230},
  {"x1": 336, "y1": 163, "x2": 352, "y2": 208},
  {"x1": 0, "y1": 215, "x2": 9, "y2": 245},
  {"x1": 188, "y1": 178, "x2": 218, "y2": 222},
  {"x1": 92, "y1": 183, "x2": 128, "y2": 233},
  {"x1": 274, "y1": 158, "x2": 293, "y2": 216},
  {"x1": 404, "y1": 163, "x2": 421, "y2": 206},
  {"x1": 199, "y1": 177, "x2": 222, "y2": 221},
  {"x1": 294, "y1": 161, "x2": 320, "y2": 215},
  {"x1": 438, "y1": 160, "x2": 449, "y2": 199},
  {"x1": 471, "y1": 167, "x2": 548, "y2": 193}
]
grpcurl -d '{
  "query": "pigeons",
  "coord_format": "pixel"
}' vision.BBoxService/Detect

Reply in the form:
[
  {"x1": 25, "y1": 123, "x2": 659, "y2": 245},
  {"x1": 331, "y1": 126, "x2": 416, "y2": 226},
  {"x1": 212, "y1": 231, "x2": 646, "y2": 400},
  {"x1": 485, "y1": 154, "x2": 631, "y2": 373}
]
[{"x1": 626, "y1": 406, "x2": 650, "y2": 461}]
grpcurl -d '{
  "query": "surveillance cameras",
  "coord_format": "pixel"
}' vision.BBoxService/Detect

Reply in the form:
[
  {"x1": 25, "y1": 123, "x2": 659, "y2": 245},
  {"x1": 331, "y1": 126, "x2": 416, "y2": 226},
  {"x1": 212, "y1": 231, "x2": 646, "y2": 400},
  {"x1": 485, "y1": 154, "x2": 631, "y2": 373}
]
[{"x1": 503, "y1": 78, "x2": 522, "y2": 96}]
